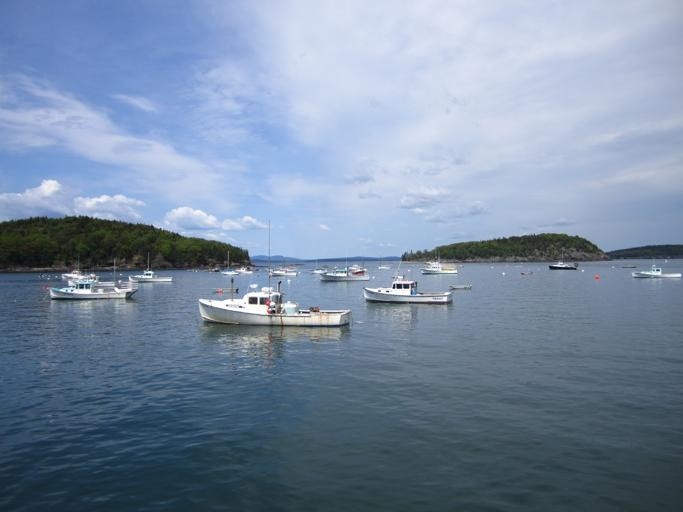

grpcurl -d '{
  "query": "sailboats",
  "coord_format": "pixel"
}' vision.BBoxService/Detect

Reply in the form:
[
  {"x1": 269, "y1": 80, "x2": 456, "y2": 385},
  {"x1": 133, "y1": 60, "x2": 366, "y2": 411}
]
[{"x1": 128, "y1": 250, "x2": 174, "y2": 283}]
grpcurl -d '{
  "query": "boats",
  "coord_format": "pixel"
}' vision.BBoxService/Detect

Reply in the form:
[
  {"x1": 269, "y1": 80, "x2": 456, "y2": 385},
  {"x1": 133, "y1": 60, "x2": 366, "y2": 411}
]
[
  {"x1": 449, "y1": 284, "x2": 472, "y2": 289},
  {"x1": 520, "y1": 271, "x2": 533, "y2": 274},
  {"x1": 50, "y1": 269, "x2": 139, "y2": 300},
  {"x1": 548, "y1": 250, "x2": 578, "y2": 270},
  {"x1": 362, "y1": 281, "x2": 453, "y2": 304},
  {"x1": 197, "y1": 286, "x2": 352, "y2": 327},
  {"x1": 419, "y1": 262, "x2": 458, "y2": 274},
  {"x1": 632, "y1": 264, "x2": 681, "y2": 278},
  {"x1": 188, "y1": 264, "x2": 391, "y2": 281}
]
